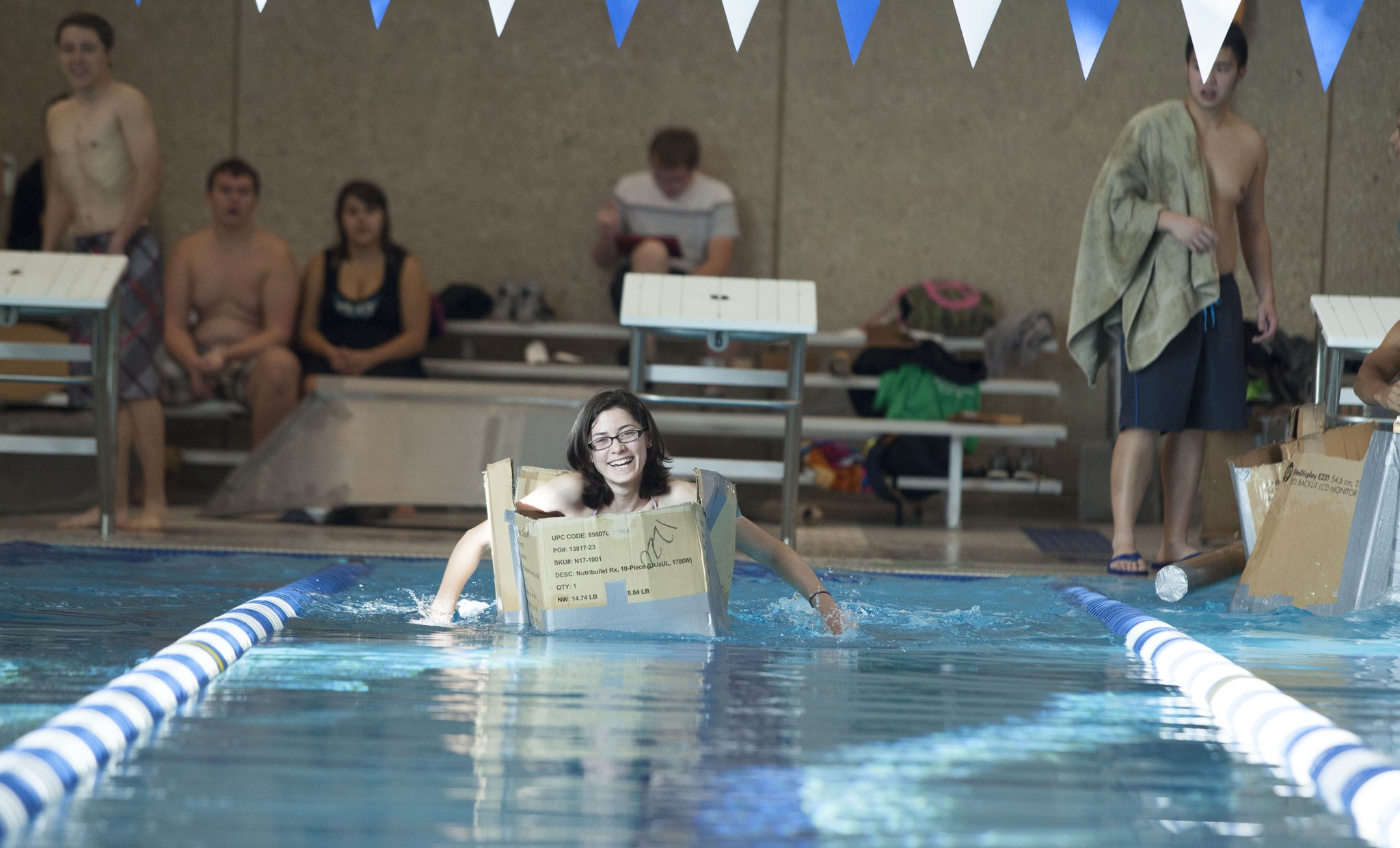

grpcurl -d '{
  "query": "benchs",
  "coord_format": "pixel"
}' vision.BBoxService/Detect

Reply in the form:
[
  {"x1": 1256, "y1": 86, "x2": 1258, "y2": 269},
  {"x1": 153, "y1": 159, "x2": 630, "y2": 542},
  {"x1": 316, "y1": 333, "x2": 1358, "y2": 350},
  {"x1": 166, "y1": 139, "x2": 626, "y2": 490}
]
[{"x1": 1, "y1": 304, "x2": 1066, "y2": 530}]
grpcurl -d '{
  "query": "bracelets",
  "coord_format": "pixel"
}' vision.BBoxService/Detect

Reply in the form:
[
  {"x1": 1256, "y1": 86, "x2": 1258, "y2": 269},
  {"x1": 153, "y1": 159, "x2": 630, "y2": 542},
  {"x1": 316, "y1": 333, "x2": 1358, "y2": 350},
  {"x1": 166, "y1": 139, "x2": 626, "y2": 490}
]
[{"x1": 809, "y1": 590, "x2": 832, "y2": 608}]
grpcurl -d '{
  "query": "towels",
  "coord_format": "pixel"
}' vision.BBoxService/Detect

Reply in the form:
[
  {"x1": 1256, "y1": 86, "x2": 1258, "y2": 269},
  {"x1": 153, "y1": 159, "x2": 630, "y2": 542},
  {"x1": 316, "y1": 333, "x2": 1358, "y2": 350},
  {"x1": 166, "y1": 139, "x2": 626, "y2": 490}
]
[{"x1": 1064, "y1": 101, "x2": 1222, "y2": 389}]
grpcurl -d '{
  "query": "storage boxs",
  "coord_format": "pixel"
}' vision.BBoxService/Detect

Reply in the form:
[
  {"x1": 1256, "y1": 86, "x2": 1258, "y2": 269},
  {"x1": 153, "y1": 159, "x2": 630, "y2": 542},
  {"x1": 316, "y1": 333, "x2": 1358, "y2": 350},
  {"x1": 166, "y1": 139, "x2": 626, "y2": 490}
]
[
  {"x1": 482, "y1": 459, "x2": 737, "y2": 640},
  {"x1": 1227, "y1": 419, "x2": 1378, "y2": 615}
]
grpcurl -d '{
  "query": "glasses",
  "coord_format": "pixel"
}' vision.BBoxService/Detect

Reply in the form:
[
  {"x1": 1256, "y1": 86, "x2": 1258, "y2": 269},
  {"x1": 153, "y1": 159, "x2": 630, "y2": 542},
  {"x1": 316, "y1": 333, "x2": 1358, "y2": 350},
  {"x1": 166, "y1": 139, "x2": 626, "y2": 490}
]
[{"x1": 587, "y1": 426, "x2": 646, "y2": 451}]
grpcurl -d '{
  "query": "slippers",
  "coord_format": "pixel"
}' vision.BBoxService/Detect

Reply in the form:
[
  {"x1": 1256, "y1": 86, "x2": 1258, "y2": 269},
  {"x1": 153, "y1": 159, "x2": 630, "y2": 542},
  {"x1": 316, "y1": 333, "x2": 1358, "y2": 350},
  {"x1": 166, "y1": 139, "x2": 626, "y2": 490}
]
[
  {"x1": 1151, "y1": 551, "x2": 1202, "y2": 570},
  {"x1": 1106, "y1": 552, "x2": 1148, "y2": 577}
]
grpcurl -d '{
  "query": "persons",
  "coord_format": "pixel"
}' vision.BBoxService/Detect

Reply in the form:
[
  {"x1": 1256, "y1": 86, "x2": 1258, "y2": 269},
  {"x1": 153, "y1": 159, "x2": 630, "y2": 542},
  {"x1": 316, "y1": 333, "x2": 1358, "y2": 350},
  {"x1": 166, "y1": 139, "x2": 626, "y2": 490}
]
[
  {"x1": 1352, "y1": 315, "x2": 1400, "y2": 412},
  {"x1": 1064, "y1": 17, "x2": 1282, "y2": 579},
  {"x1": 39, "y1": 10, "x2": 184, "y2": 533},
  {"x1": 138, "y1": 158, "x2": 307, "y2": 525},
  {"x1": 302, "y1": 173, "x2": 438, "y2": 523},
  {"x1": 423, "y1": 389, "x2": 855, "y2": 640},
  {"x1": 595, "y1": 122, "x2": 742, "y2": 368},
  {"x1": 9, "y1": 91, "x2": 74, "y2": 327}
]
